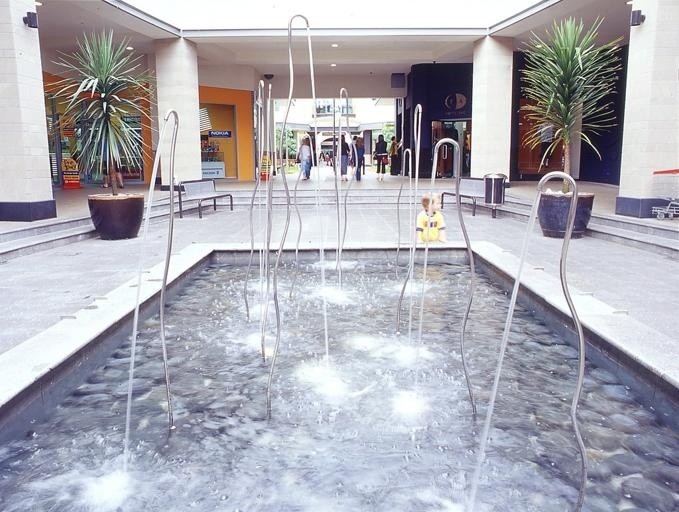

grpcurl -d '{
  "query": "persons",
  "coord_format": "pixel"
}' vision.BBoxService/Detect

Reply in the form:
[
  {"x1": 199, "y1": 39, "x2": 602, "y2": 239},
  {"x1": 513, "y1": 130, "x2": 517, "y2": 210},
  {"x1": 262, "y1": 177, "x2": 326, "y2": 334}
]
[
  {"x1": 389, "y1": 136, "x2": 403, "y2": 176},
  {"x1": 415, "y1": 197, "x2": 447, "y2": 242},
  {"x1": 373, "y1": 135, "x2": 387, "y2": 175},
  {"x1": 96, "y1": 129, "x2": 125, "y2": 188},
  {"x1": 297, "y1": 135, "x2": 364, "y2": 181}
]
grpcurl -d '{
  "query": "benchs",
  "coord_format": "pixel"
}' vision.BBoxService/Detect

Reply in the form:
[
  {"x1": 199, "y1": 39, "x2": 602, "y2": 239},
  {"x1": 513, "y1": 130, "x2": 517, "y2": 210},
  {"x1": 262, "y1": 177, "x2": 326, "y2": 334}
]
[
  {"x1": 441, "y1": 178, "x2": 496, "y2": 218},
  {"x1": 179, "y1": 179, "x2": 233, "y2": 218}
]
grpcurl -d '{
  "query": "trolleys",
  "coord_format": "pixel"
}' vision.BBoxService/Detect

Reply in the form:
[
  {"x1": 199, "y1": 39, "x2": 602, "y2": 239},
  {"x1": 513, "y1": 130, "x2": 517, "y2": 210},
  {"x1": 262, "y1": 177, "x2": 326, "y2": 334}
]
[{"x1": 650, "y1": 200, "x2": 679, "y2": 221}]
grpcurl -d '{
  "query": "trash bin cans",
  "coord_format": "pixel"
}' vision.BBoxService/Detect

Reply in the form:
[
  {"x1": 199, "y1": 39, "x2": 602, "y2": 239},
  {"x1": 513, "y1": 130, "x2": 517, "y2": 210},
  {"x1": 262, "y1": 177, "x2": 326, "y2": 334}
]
[{"x1": 483, "y1": 173, "x2": 508, "y2": 206}]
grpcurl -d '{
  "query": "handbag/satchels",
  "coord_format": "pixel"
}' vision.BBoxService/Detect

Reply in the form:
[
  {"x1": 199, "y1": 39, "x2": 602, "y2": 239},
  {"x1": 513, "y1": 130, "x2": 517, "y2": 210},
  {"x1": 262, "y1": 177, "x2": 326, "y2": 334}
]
[{"x1": 381, "y1": 156, "x2": 389, "y2": 165}]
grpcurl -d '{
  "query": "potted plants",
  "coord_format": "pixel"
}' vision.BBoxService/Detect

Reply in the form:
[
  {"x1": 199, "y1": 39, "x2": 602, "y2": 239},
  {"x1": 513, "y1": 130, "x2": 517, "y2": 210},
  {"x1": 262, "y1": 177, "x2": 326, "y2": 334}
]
[
  {"x1": 516, "y1": 15, "x2": 624, "y2": 238},
  {"x1": 44, "y1": 25, "x2": 161, "y2": 240}
]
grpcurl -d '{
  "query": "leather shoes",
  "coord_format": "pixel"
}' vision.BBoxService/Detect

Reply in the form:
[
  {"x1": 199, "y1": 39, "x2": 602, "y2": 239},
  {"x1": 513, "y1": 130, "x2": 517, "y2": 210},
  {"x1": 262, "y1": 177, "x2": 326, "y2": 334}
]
[{"x1": 299, "y1": 172, "x2": 402, "y2": 183}]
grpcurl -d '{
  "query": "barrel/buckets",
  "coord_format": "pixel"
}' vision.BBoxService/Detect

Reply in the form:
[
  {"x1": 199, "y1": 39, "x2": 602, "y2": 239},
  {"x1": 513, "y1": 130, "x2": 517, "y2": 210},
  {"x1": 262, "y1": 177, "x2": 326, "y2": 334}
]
[{"x1": 260, "y1": 164, "x2": 270, "y2": 180}]
